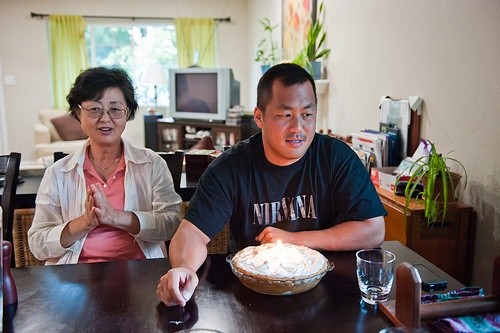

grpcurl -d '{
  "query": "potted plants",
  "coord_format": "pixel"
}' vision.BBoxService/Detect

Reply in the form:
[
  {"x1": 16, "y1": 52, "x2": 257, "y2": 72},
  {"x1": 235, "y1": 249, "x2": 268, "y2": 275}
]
[
  {"x1": 393, "y1": 138, "x2": 468, "y2": 233},
  {"x1": 255, "y1": 0, "x2": 331, "y2": 80}
]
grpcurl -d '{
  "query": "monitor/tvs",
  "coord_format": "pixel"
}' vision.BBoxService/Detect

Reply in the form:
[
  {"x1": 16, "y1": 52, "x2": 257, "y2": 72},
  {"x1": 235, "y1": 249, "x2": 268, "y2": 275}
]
[{"x1": 168, "y1": 67, "x2": 240, "y2": 122}]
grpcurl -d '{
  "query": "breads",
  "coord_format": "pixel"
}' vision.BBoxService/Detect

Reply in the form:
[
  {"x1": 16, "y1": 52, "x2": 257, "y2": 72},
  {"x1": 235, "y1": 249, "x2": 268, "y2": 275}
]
[{"x1": 233, "y1": 243, "x2": 330, "y2": 293}]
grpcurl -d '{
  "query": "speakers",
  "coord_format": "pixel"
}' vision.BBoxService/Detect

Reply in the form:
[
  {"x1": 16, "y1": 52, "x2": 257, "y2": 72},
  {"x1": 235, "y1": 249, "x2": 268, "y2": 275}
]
[
  {"x1": 143, "y1": 114, "x2": 165, "y2": 153},
  {"x1": 239, "y1": 114, "x2": 262, "y2": 141}
]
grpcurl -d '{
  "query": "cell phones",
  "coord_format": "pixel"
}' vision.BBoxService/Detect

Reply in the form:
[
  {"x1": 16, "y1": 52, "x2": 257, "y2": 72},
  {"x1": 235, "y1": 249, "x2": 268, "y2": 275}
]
[{"x1": 413, "y1": 264, "x2": 448, "y2": 290}]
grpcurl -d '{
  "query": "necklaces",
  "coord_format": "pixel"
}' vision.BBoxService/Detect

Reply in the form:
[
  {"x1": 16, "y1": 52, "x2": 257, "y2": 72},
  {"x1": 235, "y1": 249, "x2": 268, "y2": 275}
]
[{"x1": 96, "y1": 158, "x2": 118, "y2": 172}]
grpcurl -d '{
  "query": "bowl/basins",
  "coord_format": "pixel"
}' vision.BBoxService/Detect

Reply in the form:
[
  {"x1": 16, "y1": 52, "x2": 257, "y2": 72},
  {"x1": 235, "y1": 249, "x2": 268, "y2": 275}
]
[{"x1": 225, "y1": 248, "x2": 335, "y2": 295}]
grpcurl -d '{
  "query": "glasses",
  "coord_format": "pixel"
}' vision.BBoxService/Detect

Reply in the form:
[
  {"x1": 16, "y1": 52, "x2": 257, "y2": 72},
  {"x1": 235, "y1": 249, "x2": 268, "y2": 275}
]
[{"x1": 77, "y1": 103, "x2": 129, "y2": 119}]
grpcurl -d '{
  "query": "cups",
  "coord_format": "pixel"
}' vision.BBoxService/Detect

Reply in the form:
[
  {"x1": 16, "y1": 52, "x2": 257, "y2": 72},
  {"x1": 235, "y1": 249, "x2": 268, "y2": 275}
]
[{"x1": 355, "y1": 248, "x2": 398, "y2": 303}]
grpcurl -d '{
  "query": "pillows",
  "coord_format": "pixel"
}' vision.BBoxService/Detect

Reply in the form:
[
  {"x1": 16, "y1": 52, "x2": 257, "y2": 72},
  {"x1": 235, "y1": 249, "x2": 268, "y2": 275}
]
[{"x1": 51, "y1": 113, "x2": 88, "y2": 141}]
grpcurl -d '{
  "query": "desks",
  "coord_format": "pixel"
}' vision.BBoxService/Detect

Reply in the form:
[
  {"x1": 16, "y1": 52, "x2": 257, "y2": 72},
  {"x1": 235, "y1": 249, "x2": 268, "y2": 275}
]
[
  {"x1": 0, "y1": 170, "x2": 206, "y2": 209},
  {"x1": 9, "y1": 239, "x2": 462, "y2": 333}
]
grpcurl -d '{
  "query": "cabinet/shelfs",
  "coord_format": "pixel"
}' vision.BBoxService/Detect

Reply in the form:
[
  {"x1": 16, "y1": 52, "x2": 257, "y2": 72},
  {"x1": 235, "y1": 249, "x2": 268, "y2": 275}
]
[
  {"x1": 375, "y1": 182, "x2": 473, "y2": 285},
  {"x1": 157, "y1": 117, "x2": 252, "y2": 153}
]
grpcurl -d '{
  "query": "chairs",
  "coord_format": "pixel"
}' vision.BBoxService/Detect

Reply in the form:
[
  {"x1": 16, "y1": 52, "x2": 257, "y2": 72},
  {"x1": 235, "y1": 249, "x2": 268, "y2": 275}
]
[{"x1": 0, "y1": 152, "x2": 231, "y2": 267}]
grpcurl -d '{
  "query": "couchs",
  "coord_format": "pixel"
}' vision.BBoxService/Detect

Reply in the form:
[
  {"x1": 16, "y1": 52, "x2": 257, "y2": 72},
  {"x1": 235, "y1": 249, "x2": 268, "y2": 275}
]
[{"x1": 33, "y1": 108, "x2": 88, "y2": 159}]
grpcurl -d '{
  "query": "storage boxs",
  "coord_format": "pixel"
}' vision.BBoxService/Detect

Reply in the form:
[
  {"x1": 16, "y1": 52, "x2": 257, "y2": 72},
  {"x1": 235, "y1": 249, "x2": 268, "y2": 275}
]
[{"x1": 370, "y1": 166, "x2": 422, "y2": 193}]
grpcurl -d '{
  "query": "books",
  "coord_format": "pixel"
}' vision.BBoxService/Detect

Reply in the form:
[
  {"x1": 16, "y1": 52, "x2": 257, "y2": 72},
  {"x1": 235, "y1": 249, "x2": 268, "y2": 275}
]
[
  {"x1": 352, "y1": 123, "x2": 399, "y2": 167},
  {"x1": 226, "y1": 108, "x2": 255, "y2": 126}
]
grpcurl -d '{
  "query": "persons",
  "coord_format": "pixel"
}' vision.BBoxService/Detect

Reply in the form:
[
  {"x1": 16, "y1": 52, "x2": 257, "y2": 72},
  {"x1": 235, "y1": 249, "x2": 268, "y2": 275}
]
[
  {"x1": 157, "y1": 63, "x2": 389, "y2": 306},
  {"x1": 27, "y1": 67, "x2": 182, "y2": 266}
]
